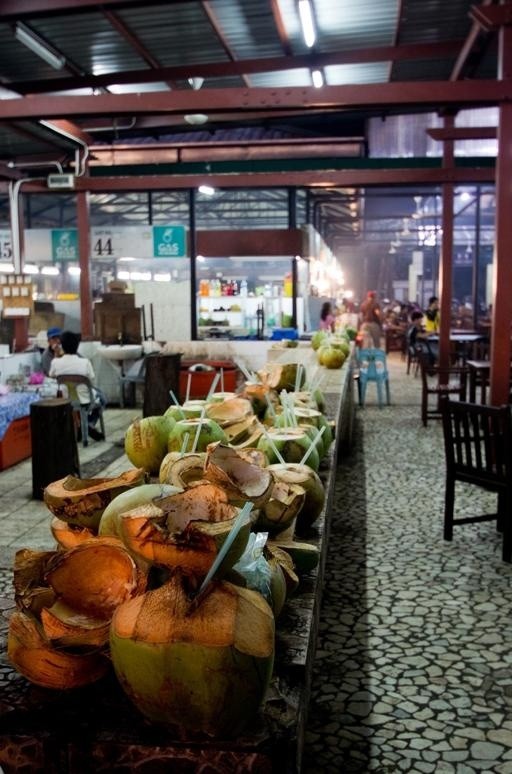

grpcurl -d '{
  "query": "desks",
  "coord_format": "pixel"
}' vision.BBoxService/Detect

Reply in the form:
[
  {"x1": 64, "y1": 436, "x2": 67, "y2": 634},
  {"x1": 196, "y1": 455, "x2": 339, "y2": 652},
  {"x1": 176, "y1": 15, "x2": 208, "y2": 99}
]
[{"x1": 96, "y1": 343, "x2": 147, "y2": 411}]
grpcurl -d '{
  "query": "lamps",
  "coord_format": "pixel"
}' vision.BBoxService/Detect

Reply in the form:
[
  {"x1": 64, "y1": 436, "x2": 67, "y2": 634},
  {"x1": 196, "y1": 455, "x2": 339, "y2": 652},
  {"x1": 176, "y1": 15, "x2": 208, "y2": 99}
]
[
  {"x1": 10, "y1": 17, "x2": 69, "y2": 75},
  {"x1": 297, "y1": 1, "x2": 319, "y2": 50}
]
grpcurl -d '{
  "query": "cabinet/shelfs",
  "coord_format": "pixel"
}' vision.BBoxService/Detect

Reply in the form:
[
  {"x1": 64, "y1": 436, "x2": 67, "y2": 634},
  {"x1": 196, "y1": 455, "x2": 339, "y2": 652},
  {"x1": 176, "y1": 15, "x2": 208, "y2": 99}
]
[
  {"x1": 0, "y1": 391, "x2": 44, "y2": 476},
  {"x1": 196, "y1": 294, "x2": 244, "y2": 331},
  {"x1": 142, "y1": 350, "x2": 186, "y2": 418}
]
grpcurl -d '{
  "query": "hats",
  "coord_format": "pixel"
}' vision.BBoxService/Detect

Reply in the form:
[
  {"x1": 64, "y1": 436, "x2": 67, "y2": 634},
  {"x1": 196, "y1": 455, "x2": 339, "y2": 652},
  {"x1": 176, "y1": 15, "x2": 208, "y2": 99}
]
[
  {"x1": 366, "y1": 290, "x2": 376, "y2": 297},
  {"x1": 46, "y1": 327, "x2": 62, "y2": 339}
]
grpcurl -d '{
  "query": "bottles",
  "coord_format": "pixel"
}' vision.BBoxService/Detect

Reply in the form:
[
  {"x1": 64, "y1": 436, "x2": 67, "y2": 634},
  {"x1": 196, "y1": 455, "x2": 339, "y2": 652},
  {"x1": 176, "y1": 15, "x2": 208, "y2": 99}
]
[
  {"x1": 283, "y1": 271, "x2": 292, "y2": 296},
  {"x1": 200, "y1": 278, "x2": 238, "y2": 296},
  {"x1": 240, "y1": 278, "x2": 248, "y2": 296},
  {"x1": 254, "y1": 285, "x2": 279, "y2": 297}
]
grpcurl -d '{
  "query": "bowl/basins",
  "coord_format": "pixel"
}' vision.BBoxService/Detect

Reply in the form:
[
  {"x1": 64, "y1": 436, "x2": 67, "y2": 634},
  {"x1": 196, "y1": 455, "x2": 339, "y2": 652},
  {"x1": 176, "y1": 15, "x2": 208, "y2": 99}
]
[{"x1": 77, "y1": 341, "x2": 143, "y2": 360}]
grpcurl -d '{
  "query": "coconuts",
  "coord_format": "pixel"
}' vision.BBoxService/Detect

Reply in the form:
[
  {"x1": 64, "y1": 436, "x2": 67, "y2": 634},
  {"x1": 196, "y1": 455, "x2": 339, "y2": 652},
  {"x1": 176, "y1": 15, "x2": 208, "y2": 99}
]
[
  {"x1": 312, "y1": 331, "x2": 350, "y2": 367},
  {"x1": 6, "y1": 361, "x2": 335, "y2": 739}
]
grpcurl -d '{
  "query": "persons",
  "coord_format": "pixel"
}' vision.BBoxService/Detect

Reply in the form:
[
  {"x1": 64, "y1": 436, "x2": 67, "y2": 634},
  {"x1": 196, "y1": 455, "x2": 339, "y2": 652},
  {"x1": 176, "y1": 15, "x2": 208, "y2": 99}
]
[
  {"x1": 319, "y1": 288, "x2": 491, "y2": 376},
  {"x1": 48, "y1": 330, "x2": 105, "y2": 443},
  {"x1": 39, "y1": 326, "x2": 83, "y2": 378}
]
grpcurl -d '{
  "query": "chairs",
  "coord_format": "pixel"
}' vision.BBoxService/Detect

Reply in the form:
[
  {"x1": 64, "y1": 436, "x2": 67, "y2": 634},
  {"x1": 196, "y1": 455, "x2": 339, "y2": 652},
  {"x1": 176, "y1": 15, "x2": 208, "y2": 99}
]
[
  {"x1": 53, "y1": 371, "x2": 111, "y2": 449},
  {"x1": 353, "y1": 346, "x2": 392, "y2": 409},
  {"x1": 394, "y1": 307, "x2": 510, "y2": 565},
  {"x1": 118, "y1": 336, "x2": 167, "y2": 410}
]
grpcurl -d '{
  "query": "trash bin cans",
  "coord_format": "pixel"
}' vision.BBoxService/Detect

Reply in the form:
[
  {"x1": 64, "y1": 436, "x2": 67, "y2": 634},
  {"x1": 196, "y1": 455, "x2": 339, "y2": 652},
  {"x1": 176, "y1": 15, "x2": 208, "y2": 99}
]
[{"x1": 178, "y1": 359, "x2": 239, "y2": 406}]
